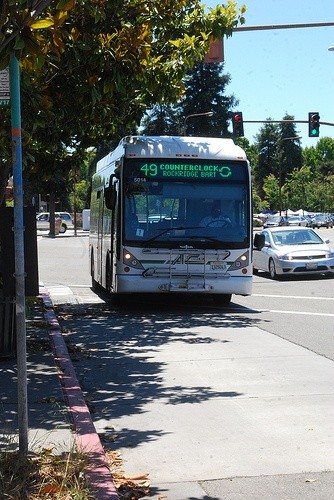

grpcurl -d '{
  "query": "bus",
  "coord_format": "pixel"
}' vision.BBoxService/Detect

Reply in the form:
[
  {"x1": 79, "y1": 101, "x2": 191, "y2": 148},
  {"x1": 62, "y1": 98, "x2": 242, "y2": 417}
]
[{"x1": 89, "y1": 135, "x2": 266, "y2": 306}]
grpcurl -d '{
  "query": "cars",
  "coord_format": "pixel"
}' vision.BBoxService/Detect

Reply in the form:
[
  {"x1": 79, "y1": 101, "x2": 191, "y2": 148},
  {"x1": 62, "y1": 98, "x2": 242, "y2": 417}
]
[
  {"x1": 288, "y1": 218, "x2": 308, "y2": 228},
  {"x1": 264, "y1": 217, "x2": 290, "y2": 228},
  {"x1": 253, "y1": 225, "x2": 334, "y2": 280},
  {"x1": 254, "y1": 214, "x2": 334, "y2": 229}
]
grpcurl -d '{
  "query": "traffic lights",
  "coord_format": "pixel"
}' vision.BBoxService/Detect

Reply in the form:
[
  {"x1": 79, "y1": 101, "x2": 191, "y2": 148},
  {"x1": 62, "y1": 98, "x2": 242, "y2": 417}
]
[
  {"x1": 308, "y1": 111, "x2": 320, "y2": 137},
  {"x1": 234, "y1": 111, "x2": 245, "y2": 138}
]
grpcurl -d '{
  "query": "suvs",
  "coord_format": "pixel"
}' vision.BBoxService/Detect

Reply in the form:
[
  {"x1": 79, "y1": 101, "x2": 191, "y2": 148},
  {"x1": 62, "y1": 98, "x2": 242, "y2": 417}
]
[{"x1": 36, "y1": 211, "x2": 74, "y2": 233}]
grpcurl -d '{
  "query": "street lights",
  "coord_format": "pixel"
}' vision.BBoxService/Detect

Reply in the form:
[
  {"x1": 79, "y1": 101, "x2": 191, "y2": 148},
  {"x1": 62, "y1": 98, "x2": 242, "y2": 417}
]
[
  {"x1": 184, "y1": 111, "x2": 214, "y2": 135},
  {"x1": 279, "y1": 136, "x2": 303, "y2": 217}
]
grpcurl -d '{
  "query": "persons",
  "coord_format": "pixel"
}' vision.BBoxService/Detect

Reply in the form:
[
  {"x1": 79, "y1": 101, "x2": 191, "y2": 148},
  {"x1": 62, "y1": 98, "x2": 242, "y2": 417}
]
[
  {"x1": 198, "y1": 206, "x2": 232, "y2": 228},
  {"x1": 55, "y1": 215, "x2": 60, "y2": 235}
]
